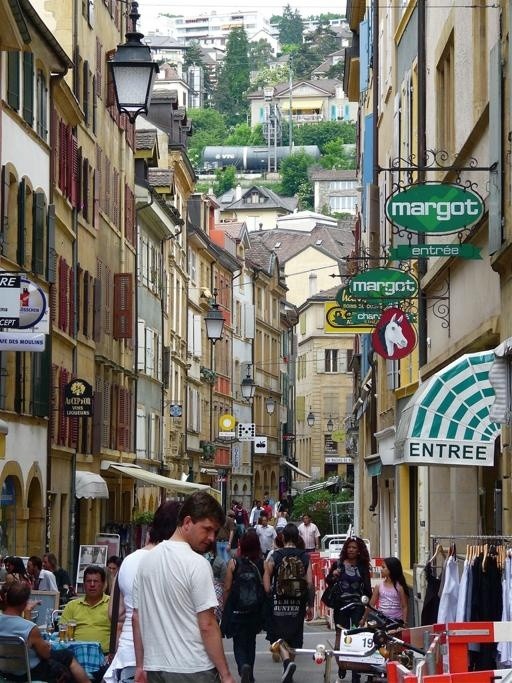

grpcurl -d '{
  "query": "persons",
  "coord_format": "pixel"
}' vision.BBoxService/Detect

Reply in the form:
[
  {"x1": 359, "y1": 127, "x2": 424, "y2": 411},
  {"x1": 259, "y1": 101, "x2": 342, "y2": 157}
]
[
  {"x1": 205, "y1": 489, "x2": 324, "y2": 682},
  {"x1": 104, "y1": 498, "x2": 183, "y2": 682},
  {"x1": 2, "y1": 553, "x2": 126, "y2": 681},
  {"x1": 131, "y1": 492, "x2": 234, "y2": 682},
  {"x1": 325, "y1": 535, "x2": 373, "y2": 682},
  {"x1": 359, "y1": 557, "x2": 409, "y2": 627}
]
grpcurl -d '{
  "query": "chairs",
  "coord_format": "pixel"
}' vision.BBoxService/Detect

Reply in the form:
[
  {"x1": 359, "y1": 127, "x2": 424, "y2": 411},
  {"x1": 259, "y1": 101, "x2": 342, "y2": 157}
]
[{"x1": 0, "y1": 596, "x2": 106, "y2": 683}]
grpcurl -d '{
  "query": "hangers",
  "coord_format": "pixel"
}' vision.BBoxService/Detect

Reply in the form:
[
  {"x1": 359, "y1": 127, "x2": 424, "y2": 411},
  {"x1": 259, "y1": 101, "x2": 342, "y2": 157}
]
[{"x1": 424, "y1": 535, "x2": 507, "y2": 574}]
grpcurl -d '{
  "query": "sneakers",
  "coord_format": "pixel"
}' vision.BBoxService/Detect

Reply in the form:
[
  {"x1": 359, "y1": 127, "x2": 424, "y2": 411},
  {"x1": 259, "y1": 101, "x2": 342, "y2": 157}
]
[
  {"x1": 240, "y1": 663, "x2": 253, "y2": 682},
  {"x1": 280, "y1": 658, "x2": 296, "y2": 683}
]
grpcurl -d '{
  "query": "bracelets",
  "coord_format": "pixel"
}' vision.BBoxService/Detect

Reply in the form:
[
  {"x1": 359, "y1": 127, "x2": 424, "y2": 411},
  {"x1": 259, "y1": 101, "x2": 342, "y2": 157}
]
[
  {"x1": 362, "y1": 614, "x2": 367, "y2": 620},
  {"x1": 403, "y1": 619, "x2": 408, "y2": 623}
]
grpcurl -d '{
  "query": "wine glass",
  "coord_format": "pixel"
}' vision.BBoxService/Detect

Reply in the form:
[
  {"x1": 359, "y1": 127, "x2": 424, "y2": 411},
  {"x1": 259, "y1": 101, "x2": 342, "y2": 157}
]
[{"x1": 45, "y1": 622, "x2": 55, "y2": 642}]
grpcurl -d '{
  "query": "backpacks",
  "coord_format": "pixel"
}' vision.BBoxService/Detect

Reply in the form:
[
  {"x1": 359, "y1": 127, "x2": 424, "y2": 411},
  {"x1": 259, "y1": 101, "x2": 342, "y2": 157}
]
[{"x1": 274, "y1": 549, "x2": 308, "y2": 599}]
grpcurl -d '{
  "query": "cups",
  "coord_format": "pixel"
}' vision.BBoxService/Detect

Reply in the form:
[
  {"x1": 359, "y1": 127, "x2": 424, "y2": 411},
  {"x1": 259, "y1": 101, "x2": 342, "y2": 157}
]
[
  {"x1": 59, "y1": 626, "x2": 67, "y2": 643},
  {"x1": 68, "y1": 623, "x2": 77, "y2": 641}
]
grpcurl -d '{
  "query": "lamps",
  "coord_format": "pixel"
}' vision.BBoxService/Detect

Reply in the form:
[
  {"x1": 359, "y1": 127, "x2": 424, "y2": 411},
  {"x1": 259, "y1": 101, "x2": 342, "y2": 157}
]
[
  {"x1": 326, "y1": 414, "x2": 333, "y2": 432},
  {"x1": 204, "y1": 288, "x2": 226, "y2": 347},
  {"x1": 264, "y1": 388, "x2": 275, "y2": 415},
  {"x1": 107, "y1": 2, "x2": 161, "y2": 122},
  {"x1": 240, "y1": 364, "x2": 257, "y2": 401},
  {"x1": 307, "y1": 406, "x2": 315, "y2": 427}
]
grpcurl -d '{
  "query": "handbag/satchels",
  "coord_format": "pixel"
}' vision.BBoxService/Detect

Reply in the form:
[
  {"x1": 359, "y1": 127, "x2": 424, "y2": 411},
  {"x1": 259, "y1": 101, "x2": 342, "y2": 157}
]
[{"x1": 321, "y1": 582, "x2": 343, "y2": 608}]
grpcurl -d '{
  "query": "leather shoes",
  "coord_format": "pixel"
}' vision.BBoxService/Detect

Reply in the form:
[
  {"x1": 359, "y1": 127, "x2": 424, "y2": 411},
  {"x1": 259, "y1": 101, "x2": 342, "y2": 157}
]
[{"x1": 338, "y1": 668, "x2": 346, "y2": 679}]
